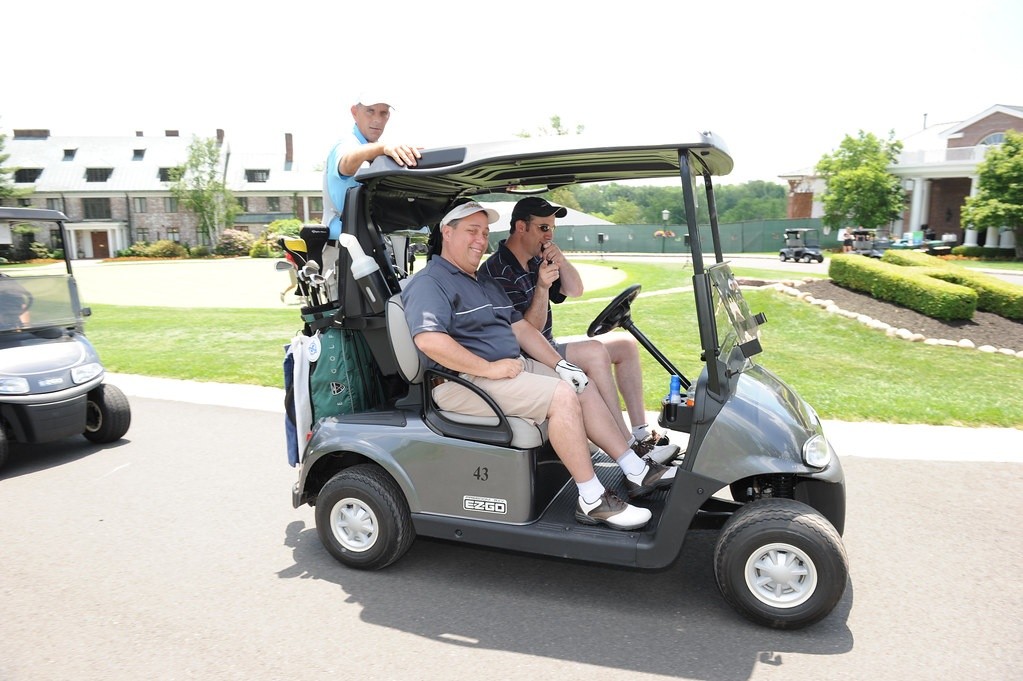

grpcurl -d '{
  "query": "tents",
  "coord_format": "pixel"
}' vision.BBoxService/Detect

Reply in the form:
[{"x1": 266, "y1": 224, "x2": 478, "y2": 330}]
[{"x1": 395, "y1": 193, "x2": 616, "y2": 250}]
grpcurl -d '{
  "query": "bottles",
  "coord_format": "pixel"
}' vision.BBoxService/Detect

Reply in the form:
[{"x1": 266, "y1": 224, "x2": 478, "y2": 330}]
[
  {"x1": 686, "y1": 379, "x2": 697, "y2": 405},
  {"x1": 669, "y1": 375, "x2": 681, "y2": 403}
]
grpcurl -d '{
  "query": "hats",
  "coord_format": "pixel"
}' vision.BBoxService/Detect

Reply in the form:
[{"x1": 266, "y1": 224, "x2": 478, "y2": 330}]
[
  {"x1": 512, "y1": 197, "x2": 568, "y2": 223},
  {"x1": 347, "y1": 86, "x2": 397, "y2": 113},
  {"x1": 439, "y1": 200, "x2": 500, "y2": 230}
]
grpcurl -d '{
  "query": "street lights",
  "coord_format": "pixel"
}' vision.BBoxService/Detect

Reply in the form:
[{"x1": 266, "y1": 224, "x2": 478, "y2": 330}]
[{"x1": 660, "y1": 209, "x2": 671, "y2": 253}]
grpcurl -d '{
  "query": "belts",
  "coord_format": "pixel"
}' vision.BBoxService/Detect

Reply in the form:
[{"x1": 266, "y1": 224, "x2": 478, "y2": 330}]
[{"x1": 431, "y1": 370, "x2": 459, "y2": 389}]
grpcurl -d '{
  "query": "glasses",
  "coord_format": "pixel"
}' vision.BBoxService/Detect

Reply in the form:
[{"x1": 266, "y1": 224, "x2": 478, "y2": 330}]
[{"x1": 527, "y1": 220, "x2": 556, "y2": 234}]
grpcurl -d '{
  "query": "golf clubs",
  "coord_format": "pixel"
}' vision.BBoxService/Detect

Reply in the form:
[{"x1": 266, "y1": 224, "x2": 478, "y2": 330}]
[{"x1": 275, "y1": 259, "x2": 332, "y2": 307}]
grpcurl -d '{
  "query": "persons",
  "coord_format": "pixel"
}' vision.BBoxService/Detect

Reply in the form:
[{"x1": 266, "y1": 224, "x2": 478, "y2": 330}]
[
  {"x1": 854, "y1": 225, "x2": 870, "y2": 241},
  {"x1": 321, "y1": 91, "x2": 424, "y2": 300},
  {"x1": 843, "y1": 226, "x2": 855, "y2": 253},
  {"x1": 402, "y1": 200, "x2": 679, "y2": 530},
  {"x1": 478, "y1": 197, "x2": 680, "y2": 464}
]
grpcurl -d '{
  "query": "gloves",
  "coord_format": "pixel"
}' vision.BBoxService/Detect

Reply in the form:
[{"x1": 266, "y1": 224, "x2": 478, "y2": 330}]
[{"x1": 555, "y1": 359, "x2": 589, "y2": 393}]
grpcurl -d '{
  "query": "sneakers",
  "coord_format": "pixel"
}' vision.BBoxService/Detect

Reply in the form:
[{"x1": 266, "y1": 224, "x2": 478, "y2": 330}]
[
  {"x1": 642, "y1": 431, "x2": 669, "y2": 446},
  {"x1": 575, "y1": 488, "x2": 654, "y2": 529},
  {"x1": 631, "y1": 435, "x2": 681, "y2": 464},
  {"x1": 625, "y1": 455, "x2": 680, "y2": 500}
]
